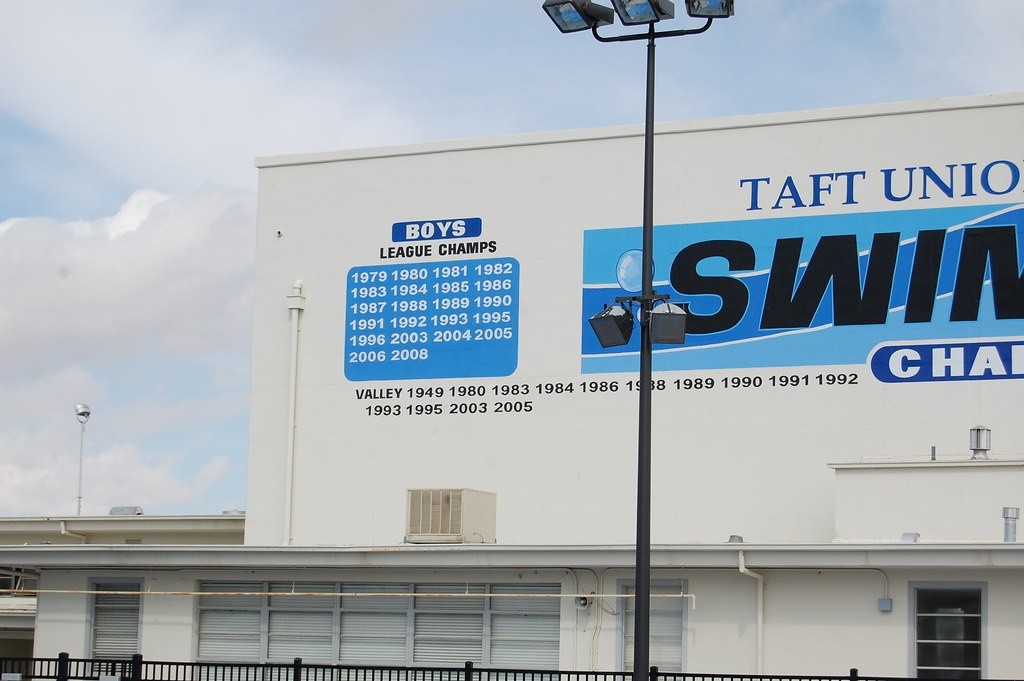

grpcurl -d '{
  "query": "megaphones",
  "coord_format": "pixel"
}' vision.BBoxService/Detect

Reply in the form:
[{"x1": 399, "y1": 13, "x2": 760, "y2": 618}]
[{"x1": 575, "y1": 596, "x2": 592, "y2": 609}]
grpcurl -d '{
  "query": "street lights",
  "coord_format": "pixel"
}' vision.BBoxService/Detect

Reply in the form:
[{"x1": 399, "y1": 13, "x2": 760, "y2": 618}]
[
  {"x1": 543, "y1": 0, "x2": 733, "y2": 681},
  {"x1": 74, "y1": 404, "x2": 90, "y2": 516}
]
